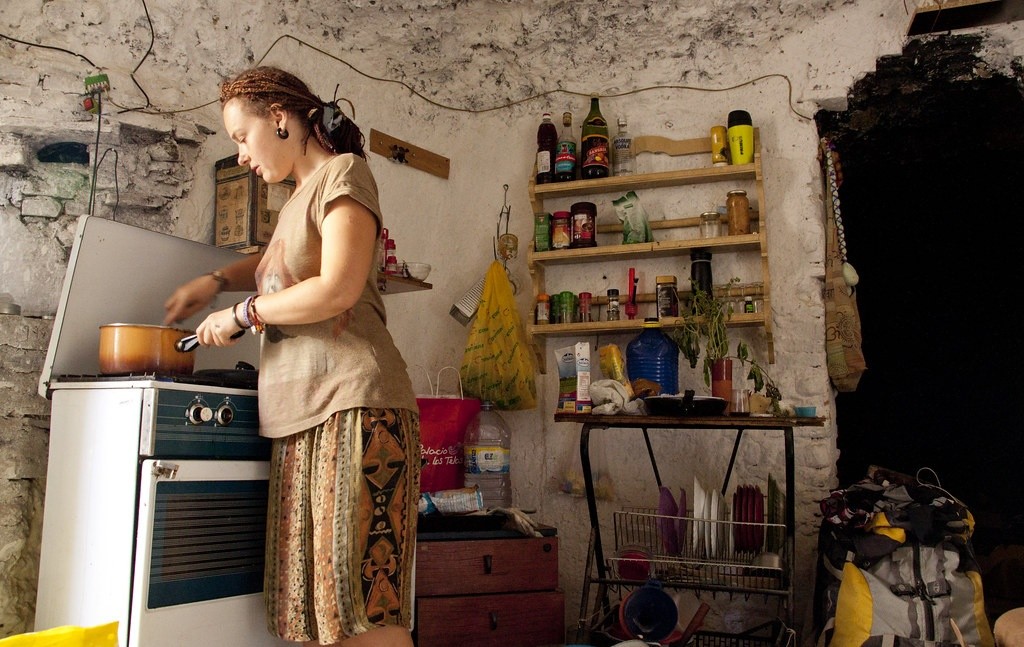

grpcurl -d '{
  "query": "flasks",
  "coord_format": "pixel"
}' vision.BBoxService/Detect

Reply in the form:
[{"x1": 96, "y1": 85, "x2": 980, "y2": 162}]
[
  {"x1": 728, "y1": 110, "x2": 754, "y2": 165},
  {"x1": 689, "y1": 251, "x2": 713, "y2": 316}
]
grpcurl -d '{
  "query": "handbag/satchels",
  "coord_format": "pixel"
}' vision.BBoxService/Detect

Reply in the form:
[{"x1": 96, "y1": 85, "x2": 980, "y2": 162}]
[
  {"x1": 406, "y1": 364, "x2": 481, "y2": 493},
  {"x1": 459, "y1": 257, "x2": 537, "y2": 410}
]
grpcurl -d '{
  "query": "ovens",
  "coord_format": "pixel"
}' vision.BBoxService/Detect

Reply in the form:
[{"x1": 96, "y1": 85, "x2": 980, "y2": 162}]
[{"x1": 32, "y1": 461, "x2": 304, "y2": 647}]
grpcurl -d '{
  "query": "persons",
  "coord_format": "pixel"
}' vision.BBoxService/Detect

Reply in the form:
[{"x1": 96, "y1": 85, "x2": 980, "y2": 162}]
[{"x1": 163, "y1": 66, "x2": 420, "y2": 647}]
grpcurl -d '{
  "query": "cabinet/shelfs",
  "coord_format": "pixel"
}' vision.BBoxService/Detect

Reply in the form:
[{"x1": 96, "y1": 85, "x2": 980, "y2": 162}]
[
  {"x1": 410, "y1": 515, "x2": 566, "y2": 647},
  {"x1": 526, "y1": 135, "x2": 776, "y2": 376}
]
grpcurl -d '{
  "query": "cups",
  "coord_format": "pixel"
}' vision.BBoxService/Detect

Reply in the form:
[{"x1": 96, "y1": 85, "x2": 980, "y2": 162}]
[
  {"x1": 710, "y1": 126, "x2": 729, "y2": 167},
  {"x1": 730, "y1": 384, "x2": 750, "y2": 418}
]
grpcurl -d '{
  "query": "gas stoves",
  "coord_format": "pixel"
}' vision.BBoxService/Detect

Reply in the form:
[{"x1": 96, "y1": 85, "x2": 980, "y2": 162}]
[{"x1": 49, "y1": 360, "x2": 273, "y2": 459}]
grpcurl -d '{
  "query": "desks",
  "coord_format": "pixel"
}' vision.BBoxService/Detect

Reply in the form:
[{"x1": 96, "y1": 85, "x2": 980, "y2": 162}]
[{"x1": 552, "y1": 412, "x2": 824, "y2": 647}]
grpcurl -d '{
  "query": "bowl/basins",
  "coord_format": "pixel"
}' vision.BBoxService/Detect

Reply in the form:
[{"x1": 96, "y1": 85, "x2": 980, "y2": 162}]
[
  {"x1": 795, "y1": 406, "x2": 816, "y2": 418},
  {"x1": 618, "y1": 587, "x2": 679, "y2": 642}
]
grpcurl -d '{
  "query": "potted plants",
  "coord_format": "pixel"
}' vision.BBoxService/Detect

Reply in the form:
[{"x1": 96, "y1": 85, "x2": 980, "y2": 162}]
[{"x1": 672, "y1": 275, "x2": 782, "y2": 416}]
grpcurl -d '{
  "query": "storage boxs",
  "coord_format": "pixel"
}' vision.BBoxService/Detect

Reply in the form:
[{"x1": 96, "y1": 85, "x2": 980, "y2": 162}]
[{"x1": 214, "y1": 154, "x2": 297, "y2": 253}]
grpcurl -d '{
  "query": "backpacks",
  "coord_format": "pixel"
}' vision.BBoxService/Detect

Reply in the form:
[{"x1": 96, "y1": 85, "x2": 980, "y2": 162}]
[{"x1": 818, "y1": 466, "x2": 976, "y2": 647}]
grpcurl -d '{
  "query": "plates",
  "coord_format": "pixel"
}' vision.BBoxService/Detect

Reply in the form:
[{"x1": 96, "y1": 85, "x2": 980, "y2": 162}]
[{"x1": 658, "y1": 475, "x2": 785, "y2": 560}]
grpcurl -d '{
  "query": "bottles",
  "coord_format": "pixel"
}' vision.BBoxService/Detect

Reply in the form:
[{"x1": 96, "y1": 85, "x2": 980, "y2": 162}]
[
  {"x1": 535, "y1": 291, "x2": 592, "y2": 325},
  {"x1": 745, "y1": 296, "x2": 753, "y2": 314},
  {"x1": 554, "y1": 112, "x2": 577, "y2": 182},
  {"x1": 606, "y1": 289, "x2": 620, "y2": 321},
  {"x1": 656, "y1": 275, "x2": 678, "y2": 318},
  {"x1": 461, "y1": 401, "x2": 512, "y2": 510},
  {"x1": 550, "y1": 210, "x2": 570, "y2": 250},
  {"x1": 536, "y1": 113, "x2": 558, "y2": 184},
  {"x1": 611, "y1": 117, "x2": 638, "y2": 176},
  {"x1": 699, "y1": 211, "x2": 722, "y2": 239},
  {"x1": 580, "y1": 92, "x2": 609, "y2": 179},
  {"x1": 625, "y1": 318, "x2": 679, "y2": 399},
  {"x1": 570, "y1": 201, "x2": 597, "y2": 248},
  {"x1": 726, "y1": 189, "x2": 750, "y2": 235}
]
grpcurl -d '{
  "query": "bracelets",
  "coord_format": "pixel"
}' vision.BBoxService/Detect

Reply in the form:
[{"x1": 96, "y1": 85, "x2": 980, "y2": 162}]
[
  {"x1": 251, "y1": 295, "x2": 264, "y2": 334},
  {"x1": 242, "y1": 296, "x2": 253, "y2": 327},
  {"x1": 233, "y1": 302, "x2": 251, "y2": 329},
  {"x1": 207, "y1": 271, "x2": 230, "y2": 291}
]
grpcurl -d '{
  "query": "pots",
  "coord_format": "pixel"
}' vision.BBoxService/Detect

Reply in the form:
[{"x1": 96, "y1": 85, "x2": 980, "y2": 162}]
[
  {"x1": 97, "y1": 322, "x2": 242, "y2": 378},
  {"x1": 644, "y1": 389, "x2": 729, "y2": 417}
]
